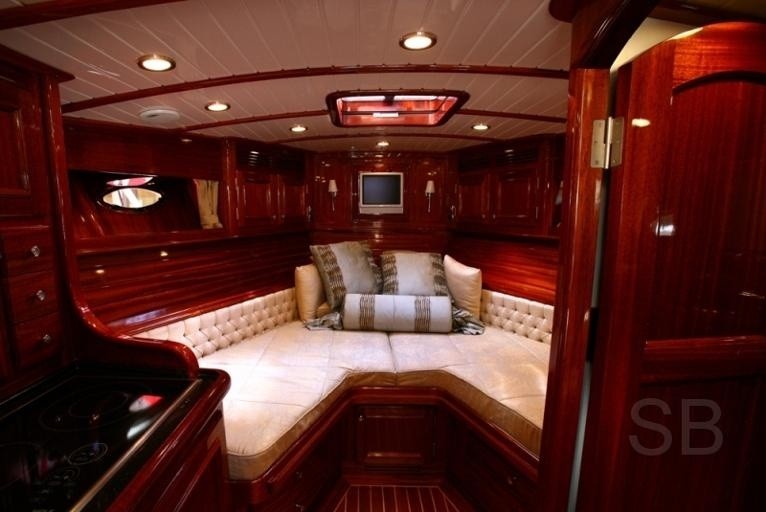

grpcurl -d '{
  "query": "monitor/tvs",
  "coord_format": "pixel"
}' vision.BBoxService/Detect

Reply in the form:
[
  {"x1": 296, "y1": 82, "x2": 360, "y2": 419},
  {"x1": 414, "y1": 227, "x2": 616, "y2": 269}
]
[{"x1": 358, "y1": 171, "x2": 404, "y2": 215}]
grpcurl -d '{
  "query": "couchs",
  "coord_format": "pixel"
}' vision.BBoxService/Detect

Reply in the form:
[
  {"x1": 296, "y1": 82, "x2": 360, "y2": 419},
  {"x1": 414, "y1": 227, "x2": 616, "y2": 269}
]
[{"x1": 112, "y1": 284, "x2": 564, "y2": 510}]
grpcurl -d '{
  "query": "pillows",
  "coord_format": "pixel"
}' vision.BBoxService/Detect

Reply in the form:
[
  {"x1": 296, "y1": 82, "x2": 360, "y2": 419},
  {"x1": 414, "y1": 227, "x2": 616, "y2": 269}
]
[{"x1": 291, "y1": 240, "x2": 484, "y2": 334}]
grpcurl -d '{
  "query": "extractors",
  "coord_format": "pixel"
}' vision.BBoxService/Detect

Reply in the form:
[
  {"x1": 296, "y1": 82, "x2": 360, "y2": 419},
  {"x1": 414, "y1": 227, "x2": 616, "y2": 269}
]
[{"x1": 0, "y1": 368, "x2": 195, "y2": 510}]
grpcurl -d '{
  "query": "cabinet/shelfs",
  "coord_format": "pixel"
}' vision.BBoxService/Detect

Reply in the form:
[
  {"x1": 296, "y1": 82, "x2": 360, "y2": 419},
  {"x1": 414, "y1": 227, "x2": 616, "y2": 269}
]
[{"x1": 0, "y1": 47, "x2": 232, "y2": 511}]
